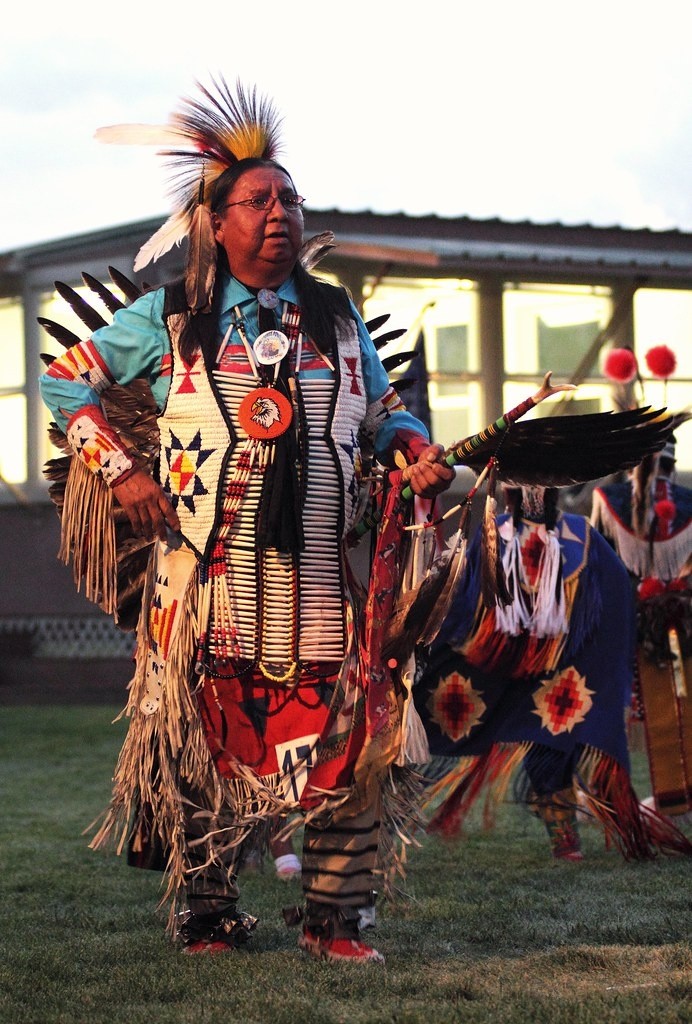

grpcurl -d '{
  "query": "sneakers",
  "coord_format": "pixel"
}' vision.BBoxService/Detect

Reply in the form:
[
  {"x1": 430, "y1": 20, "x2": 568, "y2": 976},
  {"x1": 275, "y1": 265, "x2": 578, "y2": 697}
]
[
  {"x1": 178, "y1": 919, "x2": 245, "y2": 960},
  {"x1": 302, "y1": 930, "x2": 388, "y2": 972}
]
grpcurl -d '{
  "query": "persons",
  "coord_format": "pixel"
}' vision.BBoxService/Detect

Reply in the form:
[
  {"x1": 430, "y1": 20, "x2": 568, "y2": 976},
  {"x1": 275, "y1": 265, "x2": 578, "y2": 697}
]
[
  {"x1": 593, "y1": 437, "x2": 692, "y2": 650},
  {"x1": 40, "y1": 160, "x2": 455, "y2": 961},
  {"x1": 468, "y1": 488, "x2": 648, "y2": 859}
]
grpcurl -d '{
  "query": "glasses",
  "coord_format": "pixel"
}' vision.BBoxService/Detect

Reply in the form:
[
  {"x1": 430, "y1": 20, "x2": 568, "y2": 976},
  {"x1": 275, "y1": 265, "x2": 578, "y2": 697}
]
[{"x1": 219, "y1": 194, "x2": 306, "y2": 214}]
